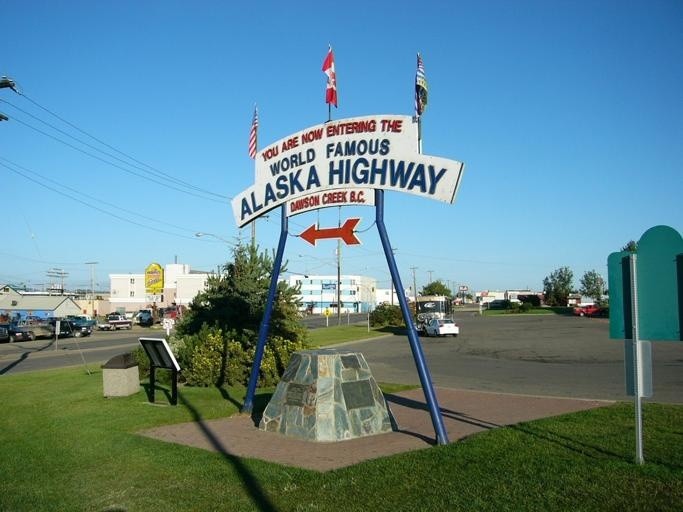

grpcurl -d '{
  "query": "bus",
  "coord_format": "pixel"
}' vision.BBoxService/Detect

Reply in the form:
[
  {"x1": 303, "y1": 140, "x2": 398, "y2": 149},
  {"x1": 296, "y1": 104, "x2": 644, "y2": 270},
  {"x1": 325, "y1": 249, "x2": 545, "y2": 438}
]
[{"x1": 416, "y1": 294, "x2": 453, "y2": 329}]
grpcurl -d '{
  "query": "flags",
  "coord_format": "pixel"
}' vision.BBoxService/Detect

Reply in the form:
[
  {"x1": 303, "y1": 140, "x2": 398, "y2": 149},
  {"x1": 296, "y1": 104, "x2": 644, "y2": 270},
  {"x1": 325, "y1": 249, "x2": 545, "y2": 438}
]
[
  {"x1": 414, "y1": 52, "x2": 427, "y2": 116},
  {"x1": 323, "y1": 47, "x2": 337, "y2": 108},
  {"x1": 249, "y1": 108, "x2": 258, "y2": 160}
]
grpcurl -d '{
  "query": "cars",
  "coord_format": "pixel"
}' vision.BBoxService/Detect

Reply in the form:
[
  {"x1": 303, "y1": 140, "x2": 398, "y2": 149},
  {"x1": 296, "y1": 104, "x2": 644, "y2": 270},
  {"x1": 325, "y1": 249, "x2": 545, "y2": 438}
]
[
  {"x1": 573, "y1": 304, "x2": 609, "y2": 318},
  {"x1": 422, "y1": 319, "x2": 459, "y2": 337}
]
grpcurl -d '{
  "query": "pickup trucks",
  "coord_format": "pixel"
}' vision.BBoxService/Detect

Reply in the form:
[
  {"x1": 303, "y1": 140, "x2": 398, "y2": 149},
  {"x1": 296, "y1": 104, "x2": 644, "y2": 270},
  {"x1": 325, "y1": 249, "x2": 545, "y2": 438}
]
[{"x1": 0, "y1": 305, "x2": 176, "y2": 343}]
